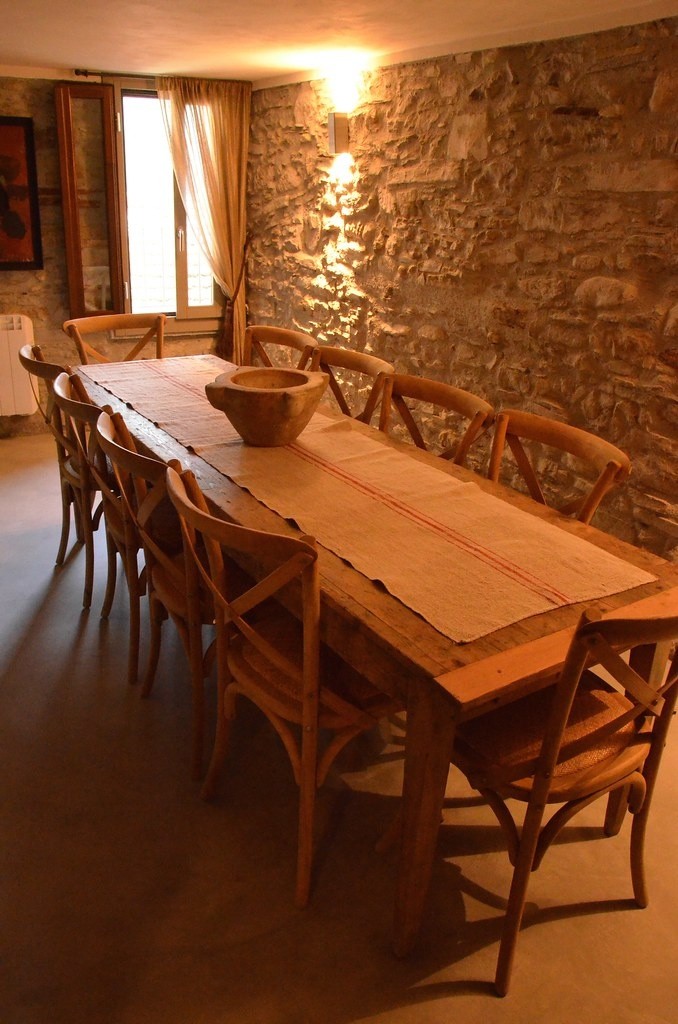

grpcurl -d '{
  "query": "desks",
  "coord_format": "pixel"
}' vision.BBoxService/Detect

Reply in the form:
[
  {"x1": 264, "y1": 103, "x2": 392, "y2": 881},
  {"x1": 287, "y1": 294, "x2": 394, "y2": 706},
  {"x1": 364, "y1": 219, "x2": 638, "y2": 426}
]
[{"x1": 66, "y1": 353, "x2": 678, "y2": 959}]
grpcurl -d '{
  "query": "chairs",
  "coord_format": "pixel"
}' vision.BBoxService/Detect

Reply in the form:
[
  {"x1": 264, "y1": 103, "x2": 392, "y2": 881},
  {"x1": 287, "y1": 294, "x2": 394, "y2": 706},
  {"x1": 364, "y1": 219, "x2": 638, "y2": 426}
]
[{"x1": 18, "y1": 313, "x2": 678, "y2": 999}]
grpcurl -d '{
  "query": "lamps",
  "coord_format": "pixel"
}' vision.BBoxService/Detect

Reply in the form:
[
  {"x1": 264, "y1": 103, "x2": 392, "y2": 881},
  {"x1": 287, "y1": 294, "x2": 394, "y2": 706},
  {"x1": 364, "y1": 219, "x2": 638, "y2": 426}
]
[{"x1": 328, "y1": 112, "x2": 349, "y2": 154}]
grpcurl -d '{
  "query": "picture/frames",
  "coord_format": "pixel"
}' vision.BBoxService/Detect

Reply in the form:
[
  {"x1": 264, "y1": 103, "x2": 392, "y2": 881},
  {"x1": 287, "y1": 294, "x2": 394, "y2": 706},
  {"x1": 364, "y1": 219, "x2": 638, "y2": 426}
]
[{"x1": 0, "y1": 116, "x2": 44, "y2": 271}]
